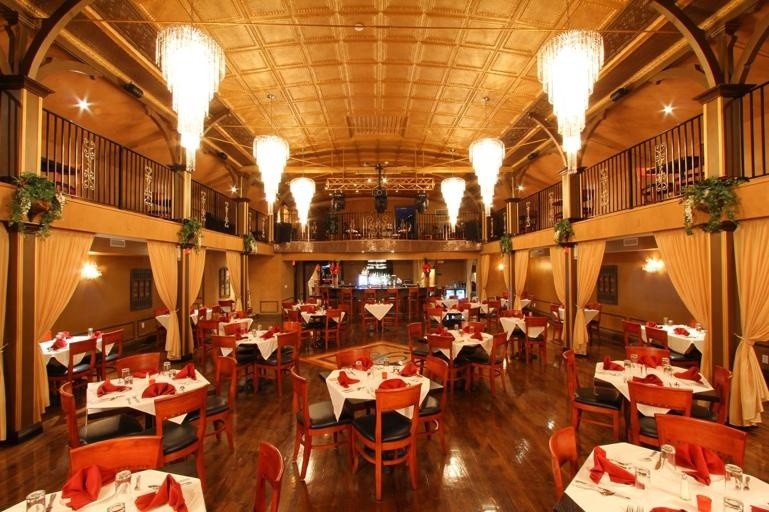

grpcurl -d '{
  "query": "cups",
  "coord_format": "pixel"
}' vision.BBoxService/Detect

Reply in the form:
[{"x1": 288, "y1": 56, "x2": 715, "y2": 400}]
[
  {"x1": 121, "y1": 361, "x2": 179, "y2": 387},
  {"x1": 108, "y1": 503, "x2": 126, "y2": 512},
  {"x1": 356, "y1": 357, "x2": 400, "y2": 380},
  {"x1": 624, "y1": 354, "x2": 679, "y2": 388},
  {"x1": 114, "y1": 470, "x2": 132, "y2": 502},
  {"x1": 635, "y1": 443, "x2": 748, "y2": 511},
  {"x1": 662, "y1": 317, "x2": 701, "y2": 334},
  {"x1": 26, "y1": 488, "x2": 46, "y2": 512}
]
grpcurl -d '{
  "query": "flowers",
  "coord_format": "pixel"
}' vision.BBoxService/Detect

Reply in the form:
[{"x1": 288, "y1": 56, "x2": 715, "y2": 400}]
[
  {"x1": 676, "y1": 176, "x2": 747, "y2": 236},
  {"x1": 177, "y1": 217, "x2": 201, "y2": 256},
  {"x1": 552, "y1": 219, "x2": 574, "y2": 245},
  {"x1": 8, "y1": 169, "x2": 66, "y2": 239}
]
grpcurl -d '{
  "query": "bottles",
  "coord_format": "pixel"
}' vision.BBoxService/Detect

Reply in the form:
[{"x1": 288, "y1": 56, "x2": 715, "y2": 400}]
[{"x1": 88, "y1": 328, "x2": 93, "y2": 339}]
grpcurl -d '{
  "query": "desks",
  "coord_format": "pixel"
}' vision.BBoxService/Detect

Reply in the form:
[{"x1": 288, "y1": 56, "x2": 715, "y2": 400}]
[
  {"x1": 552, "y1": 442, "x2": 769, "y2": 512},
  {"x1": 38, "y1": 333, "x2": 115, "y2": 374},
  {"x1": 0, "y1": 469, "x2": 207, "y2": 512},
  {"x1": 646, "y1": 155, "x2": 704, "y2": 195}
]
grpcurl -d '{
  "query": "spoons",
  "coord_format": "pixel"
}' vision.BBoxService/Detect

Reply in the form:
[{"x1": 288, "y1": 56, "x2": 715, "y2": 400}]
[{"x1": 148, "y1": 481, "x2": 193, "y2": 489}]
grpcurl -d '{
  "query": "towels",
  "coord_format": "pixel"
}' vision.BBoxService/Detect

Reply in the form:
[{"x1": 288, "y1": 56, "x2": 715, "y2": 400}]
[{"x1": 750, "y1": 506, "x2": 769, "y2": 512}]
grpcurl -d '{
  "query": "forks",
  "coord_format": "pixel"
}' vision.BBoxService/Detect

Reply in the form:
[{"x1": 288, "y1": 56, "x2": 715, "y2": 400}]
[
  {"x1": 126, "y1": 394, "x2": 140, "y2": 404},
  {"x1": 644, "y1": 450, "x2": 657, "y2": 461}
]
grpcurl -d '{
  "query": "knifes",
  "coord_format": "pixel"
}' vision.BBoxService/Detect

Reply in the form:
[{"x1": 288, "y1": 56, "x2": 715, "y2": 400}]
[{"x1": 574, "y1": 480, "x2": 631, "y2": 500}]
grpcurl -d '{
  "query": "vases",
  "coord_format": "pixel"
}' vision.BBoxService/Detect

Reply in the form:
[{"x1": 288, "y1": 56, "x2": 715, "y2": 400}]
[
  {"x1": 27, "y1": 197, "x2": 49, "y2": 213},
  {"x1": 695, "y1": 199, "x2": 724, "y2": 213},
  {"x1": 187, "y1": 232, "x2": 195, "y2": 241}
]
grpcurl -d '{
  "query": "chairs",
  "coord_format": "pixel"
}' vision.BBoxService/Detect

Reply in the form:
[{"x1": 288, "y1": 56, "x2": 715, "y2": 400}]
[
  {"x1": 637, "y1": 167, "x2": 673, "y2": 205},
  {"x1": 674, "y1": 143, "x2": 704, "y2": 196}
]
